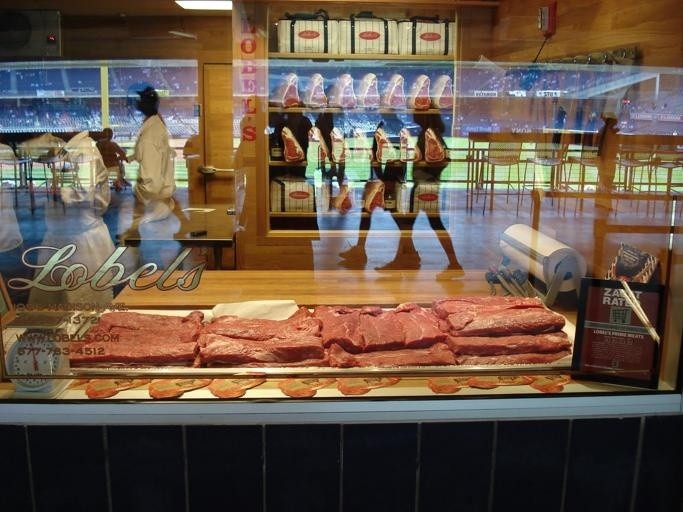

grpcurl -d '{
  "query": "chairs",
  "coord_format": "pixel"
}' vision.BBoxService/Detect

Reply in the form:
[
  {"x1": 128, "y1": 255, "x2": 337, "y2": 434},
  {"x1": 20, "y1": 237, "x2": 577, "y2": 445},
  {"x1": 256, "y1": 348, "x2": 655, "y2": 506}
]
[
  {"x1": 1, "y1": 132, "x2": 126, "y2": 217},
  {"x1": 466, "y1": 113, "x2": 683, "y2": 218}
]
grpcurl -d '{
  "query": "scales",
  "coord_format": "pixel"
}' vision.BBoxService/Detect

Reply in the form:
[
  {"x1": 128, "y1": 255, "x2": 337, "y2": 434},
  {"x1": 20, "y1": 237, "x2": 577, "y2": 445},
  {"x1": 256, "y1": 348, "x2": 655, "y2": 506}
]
[{"x1": 5, "y1": 312, "x2": 75, "y2": 399}]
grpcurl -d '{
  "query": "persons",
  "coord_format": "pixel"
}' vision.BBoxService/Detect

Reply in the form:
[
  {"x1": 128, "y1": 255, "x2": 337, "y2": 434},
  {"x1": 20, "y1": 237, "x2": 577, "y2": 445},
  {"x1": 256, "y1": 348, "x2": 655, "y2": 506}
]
[
  {"x1": 234, "y1": 96, "x2": 345, "y2": 227},
  {"x1": 375, "y1": 96, "x2": 465, "y2": 280},
  {"x1": 338, "y1": 93, "x2": 421, "y2": 262},
  {"x1": 115, "y1": 87, "x2": 190, "y2": 241},
  {"x1": 96, "y1": 128, "x2": 132, "y2": 186},
  {"x1": 1, "y1": 129, "x2": 114, "y2": 305}
]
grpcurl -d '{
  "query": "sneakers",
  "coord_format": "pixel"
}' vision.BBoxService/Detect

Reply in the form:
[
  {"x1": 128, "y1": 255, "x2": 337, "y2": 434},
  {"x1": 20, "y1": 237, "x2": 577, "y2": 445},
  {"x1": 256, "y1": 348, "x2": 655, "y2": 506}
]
[
  {"x1": 173, "y1": 222, "x2": 193, "y2": 240},
  {"x1": 115, "y1": 229, "x2": 141, "y2": 241},
  {"x1": 338, "y1": 247, "x2": 466, "y2": 281}
]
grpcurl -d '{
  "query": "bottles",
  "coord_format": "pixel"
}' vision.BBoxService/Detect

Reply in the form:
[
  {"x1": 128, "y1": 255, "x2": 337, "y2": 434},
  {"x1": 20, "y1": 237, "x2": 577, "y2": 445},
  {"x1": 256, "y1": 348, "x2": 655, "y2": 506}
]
[
  {"x1": 618, "y1": 99, "x2": 636, "y2": 132},
  {"x1": 384, "y1": 190, "x2": 396, "y2": 211},
  {"x1": 542, "y1": 49, "x2": 627, "y2": 91},
  {"x1": 271, "y1": 134, "x2": 284, "y2": 160}
]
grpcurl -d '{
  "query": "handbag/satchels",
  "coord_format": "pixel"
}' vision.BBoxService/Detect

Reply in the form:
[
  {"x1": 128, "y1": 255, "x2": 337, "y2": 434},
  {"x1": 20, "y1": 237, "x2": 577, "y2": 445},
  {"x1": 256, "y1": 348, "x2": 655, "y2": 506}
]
[{"x1": 270, "y1": 8, "x2": 456, "y2": 213}]
